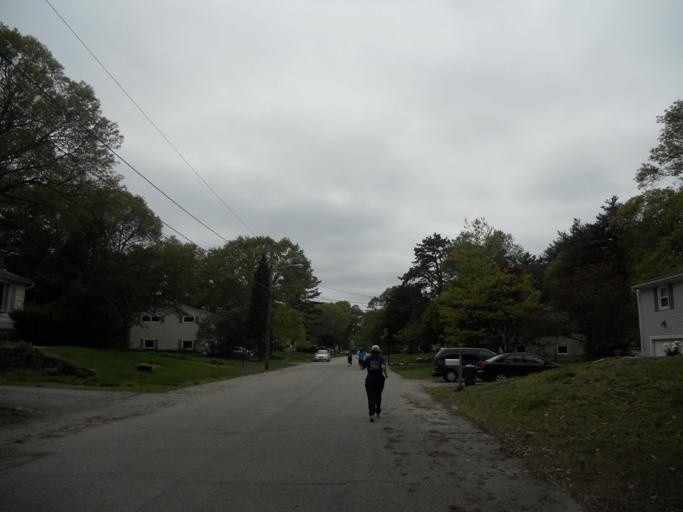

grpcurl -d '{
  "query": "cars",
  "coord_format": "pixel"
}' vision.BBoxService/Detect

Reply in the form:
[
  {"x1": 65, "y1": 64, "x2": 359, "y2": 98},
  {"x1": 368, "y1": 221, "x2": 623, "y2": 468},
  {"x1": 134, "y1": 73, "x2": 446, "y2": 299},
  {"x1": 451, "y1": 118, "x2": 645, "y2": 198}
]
[
  {"x1": 474, "y1": 351, "x2": 563, "y2": 382},
  {"x1": 339, "y1": 349, "x2": 350, "y2": 355},
  {"x1": 214, "y1": 346, "x2": 255, "y2": 360},
  {"x1": 313, "y1": 349, "x2": 330, "y2": 362}
]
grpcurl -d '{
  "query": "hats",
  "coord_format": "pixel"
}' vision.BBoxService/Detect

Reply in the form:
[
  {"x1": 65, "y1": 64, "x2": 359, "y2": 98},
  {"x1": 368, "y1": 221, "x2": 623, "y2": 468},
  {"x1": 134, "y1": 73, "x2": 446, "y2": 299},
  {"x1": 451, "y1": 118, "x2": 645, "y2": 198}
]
[{"x1": 371, "y1": 344, "x2": 381, "y2": 352}]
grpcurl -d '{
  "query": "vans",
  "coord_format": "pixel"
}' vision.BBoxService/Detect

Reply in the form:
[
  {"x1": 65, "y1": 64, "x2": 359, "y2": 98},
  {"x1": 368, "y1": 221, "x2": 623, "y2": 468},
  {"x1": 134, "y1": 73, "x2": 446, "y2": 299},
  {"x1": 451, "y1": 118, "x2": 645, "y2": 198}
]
[{"x1": 431, "y1": 346, "x2": 497, "y2": 382}]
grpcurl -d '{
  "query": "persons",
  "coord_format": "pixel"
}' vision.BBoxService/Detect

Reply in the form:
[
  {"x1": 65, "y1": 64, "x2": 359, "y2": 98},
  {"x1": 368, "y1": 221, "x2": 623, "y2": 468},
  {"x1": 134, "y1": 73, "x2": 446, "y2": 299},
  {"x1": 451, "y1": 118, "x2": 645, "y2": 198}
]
[
  {"x1": 347, "y1": 349, "x2": 353, "y2": 367},
  {"x1": 364, "y1": 344, "x2": 388, "y2": 422},
  {"x1": 357, "y1": 348, "x2": 366, "y2": 369}
]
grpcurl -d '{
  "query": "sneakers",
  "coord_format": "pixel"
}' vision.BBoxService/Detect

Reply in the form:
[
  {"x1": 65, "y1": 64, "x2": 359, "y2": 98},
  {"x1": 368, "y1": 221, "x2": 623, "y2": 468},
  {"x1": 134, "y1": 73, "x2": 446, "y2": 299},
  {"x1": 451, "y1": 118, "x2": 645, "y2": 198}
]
[{"x1": 370, "y1": 415, "x2": 375, "y2": 422}]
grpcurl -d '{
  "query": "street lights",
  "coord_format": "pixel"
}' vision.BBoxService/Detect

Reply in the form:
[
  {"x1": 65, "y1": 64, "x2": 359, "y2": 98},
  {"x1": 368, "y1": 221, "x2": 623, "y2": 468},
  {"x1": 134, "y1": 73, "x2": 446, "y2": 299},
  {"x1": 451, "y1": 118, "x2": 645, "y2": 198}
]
[{"x1": 264, "y1": 264, "x2": 304, "y2": 371}]
